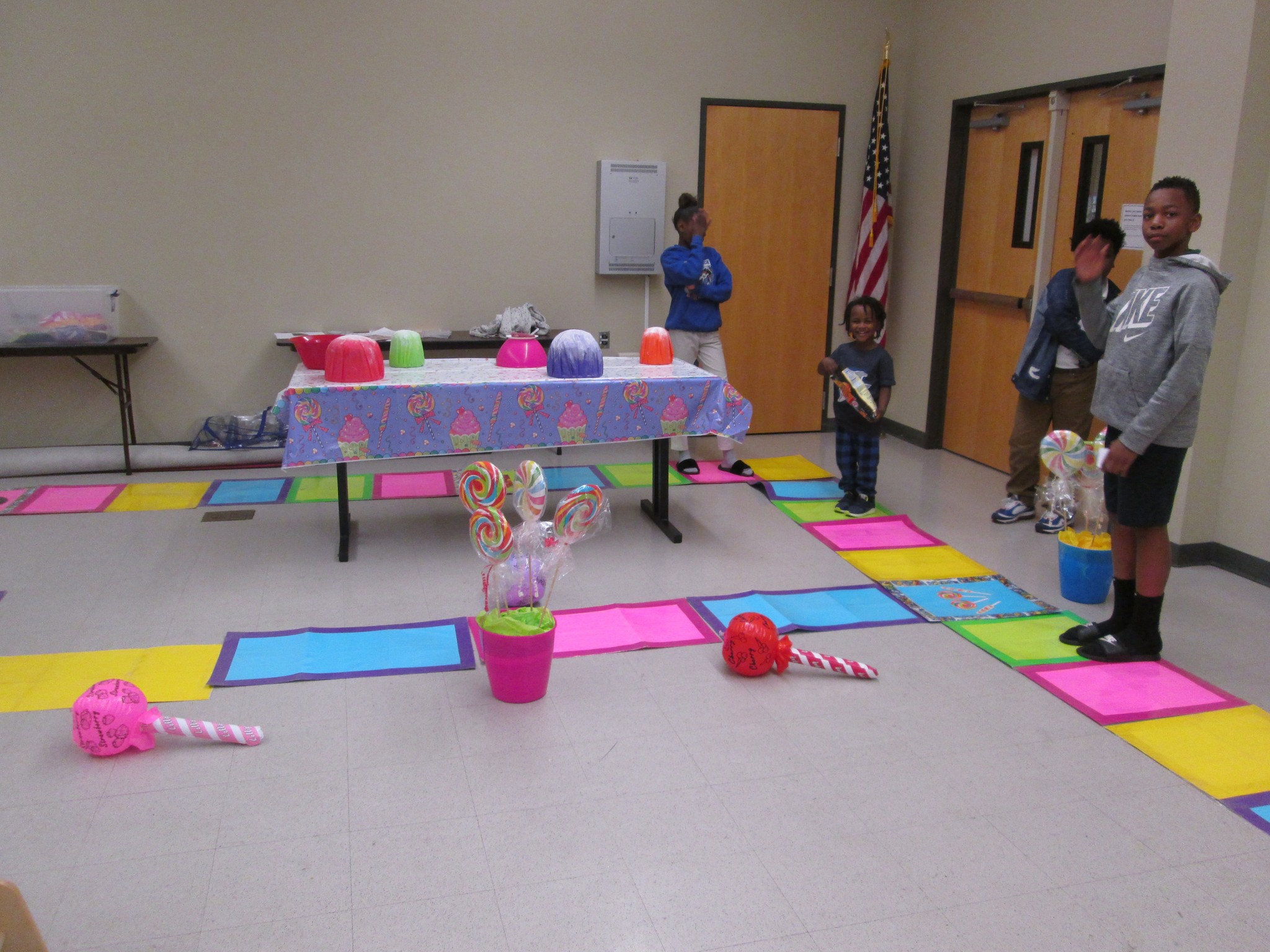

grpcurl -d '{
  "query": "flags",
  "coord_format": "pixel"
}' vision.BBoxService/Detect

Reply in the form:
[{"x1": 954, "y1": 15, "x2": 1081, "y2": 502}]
[{"x1": 847, "y1": 59, "x2": 893, "y2": 349}]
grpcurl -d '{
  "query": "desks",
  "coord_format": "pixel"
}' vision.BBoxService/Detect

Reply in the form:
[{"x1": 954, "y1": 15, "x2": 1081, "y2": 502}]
[
  {"x1": 0, "y1": 336, "x2": 157, "y2": 477},
  {"x1": 277, "y1": 327, "x2": 569, "y2": 456},
  {"x1": 279, "y1": 357, "x2": 754, "y2": 565}
]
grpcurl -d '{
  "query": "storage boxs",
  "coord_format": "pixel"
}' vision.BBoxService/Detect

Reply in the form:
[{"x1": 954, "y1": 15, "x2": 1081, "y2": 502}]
[{"x1": 0, "y1": 284, "x2": 121, "y2": 343}]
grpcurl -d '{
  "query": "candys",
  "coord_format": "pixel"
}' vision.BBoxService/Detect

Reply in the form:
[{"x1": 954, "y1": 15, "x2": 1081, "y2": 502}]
[
  {"x1": 459, "y1": 460, "x2": 603, "y2": 628},
  {"x1": 722, "y1": 612, "x2": 879, "y2": 679},
  {"x1": 1040, "y1": 427, "x2": 1110, "y2": 547},
  {"x1": 71, "y1": 680, "x2": 263, "y2": 755}
]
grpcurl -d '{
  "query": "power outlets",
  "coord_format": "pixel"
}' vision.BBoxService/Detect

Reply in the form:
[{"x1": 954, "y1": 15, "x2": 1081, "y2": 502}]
[{"x1": 599, "y1": 330, "x2": 611, "y2": 349}]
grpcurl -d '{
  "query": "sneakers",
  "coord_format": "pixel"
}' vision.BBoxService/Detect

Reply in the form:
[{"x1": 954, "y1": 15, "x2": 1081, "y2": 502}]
[
  {"x1": 834, "y1": 492, "x2": 858, "y2": 512},
  {"x1": 991, "y1": 496, "x2": 1035, "y2": 525},
  {"x1": 1035, "y1": 510, "x2": 1074, "y2": 533},
  {"x1": 846, "y1": 495, "x2": 875, "y2": 517}
]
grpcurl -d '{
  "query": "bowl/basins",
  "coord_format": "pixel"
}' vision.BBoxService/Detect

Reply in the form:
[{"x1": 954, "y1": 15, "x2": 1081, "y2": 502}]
[
  {"x1": 495, "y1": 338, "x2": 547, "y2": 368},
  {"x1": 290, "y1": 334, "x2": 346, "y2": 370}
]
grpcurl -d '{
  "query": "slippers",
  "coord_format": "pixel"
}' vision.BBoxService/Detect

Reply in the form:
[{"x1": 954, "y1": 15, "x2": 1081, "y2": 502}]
[
  {"x1": 1059, "y1": 621, "x2": 1101, "y2": 645},
  {"x1": 676, "y1": 458, "x2": 699, "y2": 474},
  {"x1": 1077, "y1": 634, "x2": 1161, "y2": 662},
  {"x1": 718, "y1": 459, "x2": 755, "y2": 477}
]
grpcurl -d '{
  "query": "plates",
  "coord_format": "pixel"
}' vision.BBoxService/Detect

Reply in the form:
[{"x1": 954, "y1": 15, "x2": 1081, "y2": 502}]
[{"x1": 506, "y1": 335, "x2": 539, "y2": 339}]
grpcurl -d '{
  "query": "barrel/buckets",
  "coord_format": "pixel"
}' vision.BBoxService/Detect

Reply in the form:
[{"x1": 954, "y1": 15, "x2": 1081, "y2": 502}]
[
  {"x1": 479, "y1": 607, "x2": 557, "y2": 704},
  {"x1": 1058, "y1": 538, "x2": 1113, "y2": 604}
]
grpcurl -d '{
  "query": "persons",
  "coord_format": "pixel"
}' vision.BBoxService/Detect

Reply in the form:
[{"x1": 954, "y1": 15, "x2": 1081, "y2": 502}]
[
  {"x1": 991, "y1": 217, "x2": 1126, "y2": 533},
  {"x1": 1058, "y1": 176, "x2": 1231, "y2": 663},
  {"x1": 660, "y1": 193, "x2": 754, "y2": 476},
  {"x1": 817, "y1": 296, "x2": 896, "y2": 518}
]
